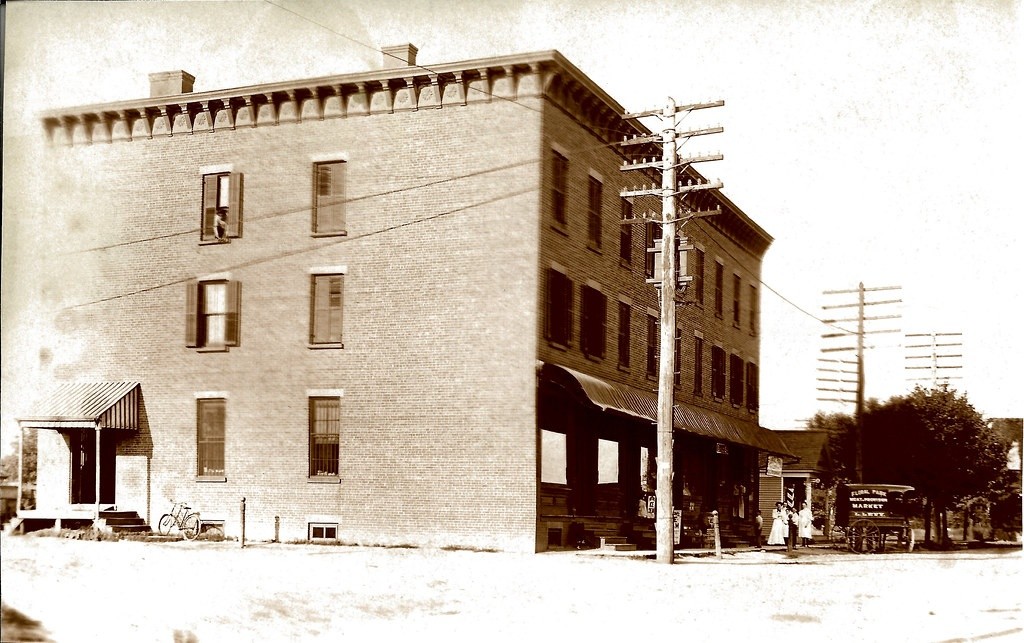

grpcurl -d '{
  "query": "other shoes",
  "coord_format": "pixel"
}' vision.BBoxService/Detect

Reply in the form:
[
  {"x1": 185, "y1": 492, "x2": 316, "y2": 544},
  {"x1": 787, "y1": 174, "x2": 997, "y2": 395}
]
[{"x1": 755, "y1": 546, "x2": 762, "y2": 548}]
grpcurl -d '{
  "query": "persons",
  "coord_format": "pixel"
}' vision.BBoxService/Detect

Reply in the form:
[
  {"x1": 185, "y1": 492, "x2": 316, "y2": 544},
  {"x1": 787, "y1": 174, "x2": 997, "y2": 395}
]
[
  {"x1": 767, "y1": 501, "x2": 812, "y2": 548},
  {"x1": 213, "y1": 209, "x2": 227, "y2": 240},
  {"x1": 751, "y1": 511, "x2": 763, "y2": 548}
]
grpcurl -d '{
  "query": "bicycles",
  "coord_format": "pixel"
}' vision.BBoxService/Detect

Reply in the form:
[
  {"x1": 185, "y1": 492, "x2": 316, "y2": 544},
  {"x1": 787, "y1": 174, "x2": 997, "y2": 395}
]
[{"x1": 158, "y1": 498, "x2": 201, "y2": 541}]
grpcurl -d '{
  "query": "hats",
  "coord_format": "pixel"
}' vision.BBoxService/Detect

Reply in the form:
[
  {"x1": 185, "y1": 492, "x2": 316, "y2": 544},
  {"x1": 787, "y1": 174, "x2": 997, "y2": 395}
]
[{"x1": 776, "y1": 502, "x2": 782, "y2": 507}]
